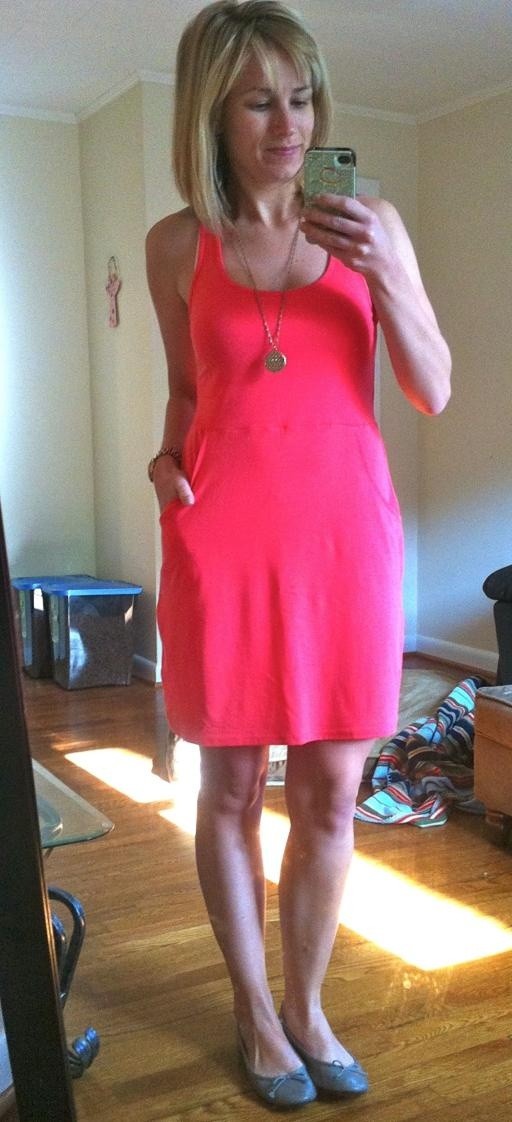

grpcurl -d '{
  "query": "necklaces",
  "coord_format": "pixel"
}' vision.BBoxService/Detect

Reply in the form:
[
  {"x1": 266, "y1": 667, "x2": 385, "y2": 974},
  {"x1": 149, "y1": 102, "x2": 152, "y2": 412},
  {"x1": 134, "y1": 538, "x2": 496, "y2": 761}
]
[{"x1": 230, "y1": 213, "x2": 304, "y2": 373}]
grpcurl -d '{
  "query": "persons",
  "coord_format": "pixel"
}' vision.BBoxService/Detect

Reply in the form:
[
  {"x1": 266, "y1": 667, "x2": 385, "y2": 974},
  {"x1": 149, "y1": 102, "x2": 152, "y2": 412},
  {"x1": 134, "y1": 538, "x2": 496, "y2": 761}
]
[{"x1": 145, "y1": 0, "x2": 453, "y2": 1108}]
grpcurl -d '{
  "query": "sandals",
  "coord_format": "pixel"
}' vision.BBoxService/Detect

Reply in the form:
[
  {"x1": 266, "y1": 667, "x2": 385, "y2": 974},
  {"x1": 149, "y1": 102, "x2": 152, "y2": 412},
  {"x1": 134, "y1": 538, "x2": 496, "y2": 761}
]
[{"x1": 238, "y1": 1000, "x2": 368, "y2": 1105}]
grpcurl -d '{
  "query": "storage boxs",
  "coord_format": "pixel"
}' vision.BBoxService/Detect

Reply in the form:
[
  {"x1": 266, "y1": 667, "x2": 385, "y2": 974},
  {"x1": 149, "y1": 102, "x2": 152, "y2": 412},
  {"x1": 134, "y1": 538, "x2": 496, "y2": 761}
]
[{"x1": 11, "y1": 573, "x2": 143, "y2": 692}]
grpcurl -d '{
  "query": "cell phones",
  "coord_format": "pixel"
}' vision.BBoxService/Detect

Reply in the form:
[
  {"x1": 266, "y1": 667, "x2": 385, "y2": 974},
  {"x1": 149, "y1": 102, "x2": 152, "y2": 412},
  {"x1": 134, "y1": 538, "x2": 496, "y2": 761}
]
[{"x1": 303, "y1": 147, "x2": 356, "y2": 245}]
